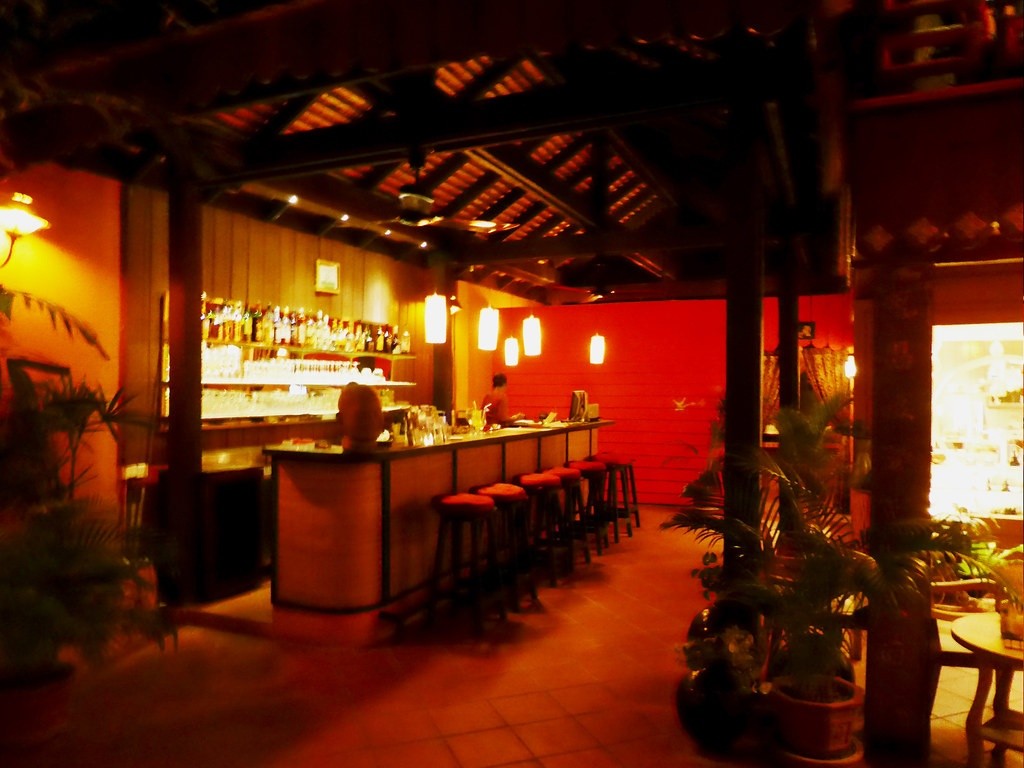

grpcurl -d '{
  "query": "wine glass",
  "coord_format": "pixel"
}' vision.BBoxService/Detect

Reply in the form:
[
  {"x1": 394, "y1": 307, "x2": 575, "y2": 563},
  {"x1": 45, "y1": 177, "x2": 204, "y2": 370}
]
[{"x1": 202, "y1": 343, "x2": 362, "y2": 417}]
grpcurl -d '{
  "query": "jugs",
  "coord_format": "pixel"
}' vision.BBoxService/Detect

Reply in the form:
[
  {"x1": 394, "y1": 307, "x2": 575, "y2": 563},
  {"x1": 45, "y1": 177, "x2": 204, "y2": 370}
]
[{"x1": 451, "y1": 407, "x2": 487, "y2": 435}]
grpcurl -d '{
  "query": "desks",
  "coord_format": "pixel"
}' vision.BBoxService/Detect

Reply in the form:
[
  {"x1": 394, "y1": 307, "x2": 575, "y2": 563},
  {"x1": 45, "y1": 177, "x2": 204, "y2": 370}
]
[{"x1": 949, "y1": 612, "x2": 1024, "y2": 768}]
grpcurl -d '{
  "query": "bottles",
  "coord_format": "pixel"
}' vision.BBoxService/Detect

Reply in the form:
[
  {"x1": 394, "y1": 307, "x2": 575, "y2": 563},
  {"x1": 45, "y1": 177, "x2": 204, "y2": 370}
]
[
  {"x1": 201, "y1": 291, "x2": 411, "y2": 355},
  {"x1": 393, "y1": 404, "x2": 450, "y2": 447}
]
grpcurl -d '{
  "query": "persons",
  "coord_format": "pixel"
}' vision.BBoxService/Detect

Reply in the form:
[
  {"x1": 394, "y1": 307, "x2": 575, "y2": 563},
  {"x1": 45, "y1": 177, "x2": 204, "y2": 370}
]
[{"x1": 480, "y1": 372, "x2": 526, "y2": 431}]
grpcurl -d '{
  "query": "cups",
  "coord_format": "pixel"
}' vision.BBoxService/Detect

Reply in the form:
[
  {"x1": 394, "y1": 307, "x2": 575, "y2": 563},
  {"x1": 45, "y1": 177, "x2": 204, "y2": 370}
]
[{"x1": 361, "y1": 367, "x2": 386, "y2": 382}]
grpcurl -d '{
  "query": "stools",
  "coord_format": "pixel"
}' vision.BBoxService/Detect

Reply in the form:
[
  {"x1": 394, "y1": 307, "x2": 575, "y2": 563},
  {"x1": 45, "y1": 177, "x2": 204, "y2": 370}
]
[{"x1": 422, "y1": 453, "x2": 642, "y2": 637}]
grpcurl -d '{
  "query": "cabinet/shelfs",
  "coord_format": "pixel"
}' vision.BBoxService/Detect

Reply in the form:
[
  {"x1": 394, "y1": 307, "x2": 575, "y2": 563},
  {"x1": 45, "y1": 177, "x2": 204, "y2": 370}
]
[{"x1": 159, "y1": 336, "x2": 417, "y2": 433}]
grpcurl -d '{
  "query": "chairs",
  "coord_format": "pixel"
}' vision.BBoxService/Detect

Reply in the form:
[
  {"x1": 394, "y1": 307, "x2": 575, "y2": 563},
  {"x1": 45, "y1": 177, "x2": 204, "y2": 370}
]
[{"x1": 929, "y1": 577, "x2": 1024, "y2": 672}]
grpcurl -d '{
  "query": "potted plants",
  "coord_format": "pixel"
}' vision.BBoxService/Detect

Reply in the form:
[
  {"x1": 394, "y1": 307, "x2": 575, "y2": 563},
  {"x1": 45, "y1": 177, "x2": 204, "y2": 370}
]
[
  {"x1": 657, "y1": 371, "x2": 1024, "y2": 758},
  {"x1": 0, "y1": 376, "x2": 180, "y2": 749}
]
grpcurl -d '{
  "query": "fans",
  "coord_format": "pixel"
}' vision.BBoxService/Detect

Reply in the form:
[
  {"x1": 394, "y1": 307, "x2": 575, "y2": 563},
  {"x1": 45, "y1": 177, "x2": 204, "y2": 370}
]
[{"x1": 331, "y1": 149, "x2": 520, "y2": 233}]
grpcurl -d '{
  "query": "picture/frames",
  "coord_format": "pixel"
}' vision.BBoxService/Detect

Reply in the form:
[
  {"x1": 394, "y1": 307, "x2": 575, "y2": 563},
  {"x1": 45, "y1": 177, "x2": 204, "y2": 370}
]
[{"x1": 315, "y1": 258, "x2": 341, "y2": 296}]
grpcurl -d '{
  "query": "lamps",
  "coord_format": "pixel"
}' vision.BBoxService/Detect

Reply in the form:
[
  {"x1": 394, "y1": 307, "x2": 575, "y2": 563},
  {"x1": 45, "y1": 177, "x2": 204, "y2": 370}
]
[{"x1": 427, "y1": 289, "x2": 605, "y2": 369}]
